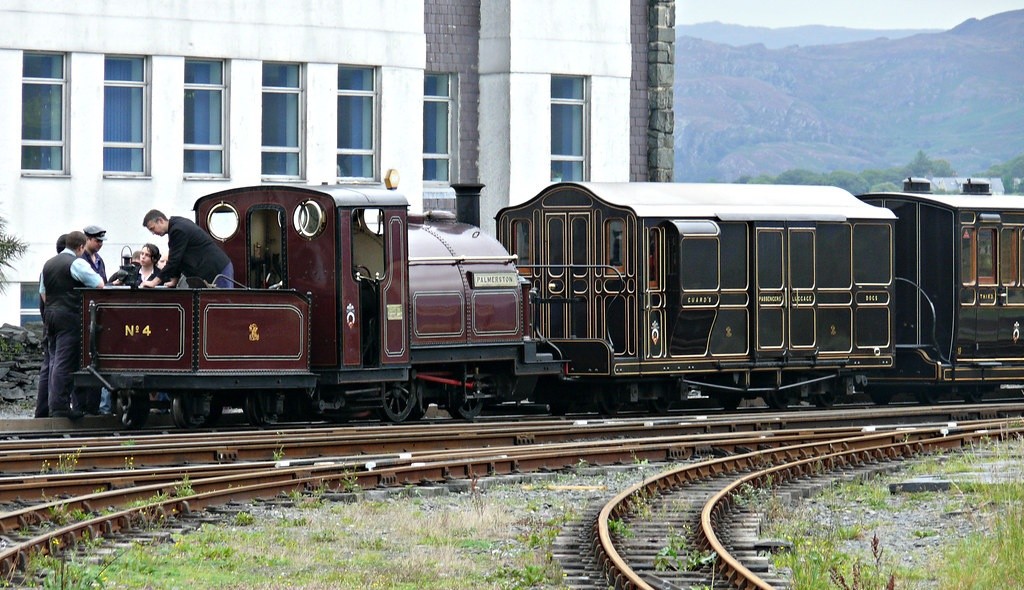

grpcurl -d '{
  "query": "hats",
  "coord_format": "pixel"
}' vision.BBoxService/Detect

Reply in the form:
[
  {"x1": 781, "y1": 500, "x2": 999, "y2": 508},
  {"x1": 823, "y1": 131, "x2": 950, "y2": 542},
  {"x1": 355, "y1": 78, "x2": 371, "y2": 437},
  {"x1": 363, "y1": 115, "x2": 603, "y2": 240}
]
[{"x1": 84, "y1": 226, "x2": 107, "y2": 241}]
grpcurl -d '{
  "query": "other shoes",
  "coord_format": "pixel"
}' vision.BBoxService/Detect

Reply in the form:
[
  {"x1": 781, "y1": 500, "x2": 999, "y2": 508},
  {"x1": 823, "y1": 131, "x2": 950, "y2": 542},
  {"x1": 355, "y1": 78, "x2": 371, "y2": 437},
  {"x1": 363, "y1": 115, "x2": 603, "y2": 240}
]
[{"x1": 49, "y1": 409, "x2": 84, "y2": 418}]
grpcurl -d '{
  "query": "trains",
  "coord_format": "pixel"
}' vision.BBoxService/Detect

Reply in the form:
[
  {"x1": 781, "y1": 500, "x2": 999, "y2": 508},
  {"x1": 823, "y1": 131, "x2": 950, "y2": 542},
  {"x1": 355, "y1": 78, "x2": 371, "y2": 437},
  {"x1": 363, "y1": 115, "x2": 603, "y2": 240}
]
[{"x1": 65, "y1": 177, "x2": 1024, "y2": 434}]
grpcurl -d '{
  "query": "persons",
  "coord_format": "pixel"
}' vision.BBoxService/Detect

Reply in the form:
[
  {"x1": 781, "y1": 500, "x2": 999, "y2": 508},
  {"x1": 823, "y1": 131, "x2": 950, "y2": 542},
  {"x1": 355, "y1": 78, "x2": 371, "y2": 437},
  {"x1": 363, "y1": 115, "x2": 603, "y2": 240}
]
[
  {"x1": 142, "y1": 209, "x2": 234, "y2": 288},
  {"x1": 34, "y1": 226, "x2": 168, "y2": 417},
  {"x1": 125, "y1": 244, "x2": 170, "y2": 288}
]
